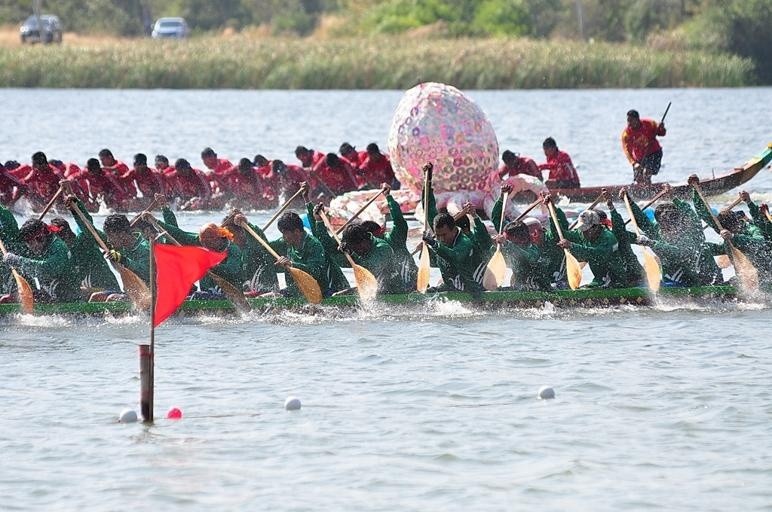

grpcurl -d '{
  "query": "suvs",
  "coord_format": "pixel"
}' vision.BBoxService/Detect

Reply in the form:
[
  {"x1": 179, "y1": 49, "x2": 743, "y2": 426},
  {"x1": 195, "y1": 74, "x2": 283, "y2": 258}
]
[
  {"x1": 20, "y1": 15, "x2": 62, "y2": 43},
  {"x1": 151, "y1": 17, "x2": 187, "y2": 40}
]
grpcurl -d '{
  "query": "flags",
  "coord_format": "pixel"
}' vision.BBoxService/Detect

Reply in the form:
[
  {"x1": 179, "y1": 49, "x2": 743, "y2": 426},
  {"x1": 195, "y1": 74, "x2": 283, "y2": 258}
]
[{"x1": 152, "y1": 242, "x2": 228, "y2": 325}]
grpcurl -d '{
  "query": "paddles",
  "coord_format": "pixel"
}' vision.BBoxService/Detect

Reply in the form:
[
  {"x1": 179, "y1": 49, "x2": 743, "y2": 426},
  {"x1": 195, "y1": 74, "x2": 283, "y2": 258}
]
[
  {"x1": 623, "y1": 191, "x2": 661, "y2": 292},
  {"x1": 69, "y1": 199, "x2": 151, "y2": 311},
  {"x1": 0, "y1": 240, "x2": 33, "y2": 314},
  {"x1": 484, "y1": 191, "x2": 507, "y2": 291},
  {"x1": 417, "y1": 168, "x2": 430, "y2": 291},
  {"x1": 239, "y1": 219, "x2": 323, "y2": 305},
  {"x1": 147, "y1": 217, "x2": 251, "y2": 313},
  {"x1": 317, "y1": 208, "x2": 378, "y2": 300},
  {"x1": 693, "y1": 180, "x2": 758, "y2": 289},
  {"x1": 547, "y1": 200, "x2": 582, "y2": 292}
]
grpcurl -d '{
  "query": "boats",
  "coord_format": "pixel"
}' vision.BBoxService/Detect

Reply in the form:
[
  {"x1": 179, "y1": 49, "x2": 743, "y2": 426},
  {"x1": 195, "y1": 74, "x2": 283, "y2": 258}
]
[
  {"x1": 0, "y1": 279, "x2": 770, "y2": 322},
  {"x1": 0, "y1": 137, "x2": 772, "y2": 212}
]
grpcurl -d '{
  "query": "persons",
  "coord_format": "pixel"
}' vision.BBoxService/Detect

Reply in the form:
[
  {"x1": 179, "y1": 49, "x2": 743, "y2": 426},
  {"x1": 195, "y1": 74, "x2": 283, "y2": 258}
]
[
  {"x1": 3, "y1": 151, "x2": 69, "y2": 214},
  {"x1": 299, "y1": 180, "x2": 418, "y2": 296},
  {"x1": 59, "y1": 158, "x2": 128, "y2": 213},
  {"x1": 0, "y1": 219, "x2": 82, "y2": 304},
  {"x1": 141, "y1": 212, "x2": 248, "y2": 301},
  {"x1": 155, "y1": 155, "x2": 180, "y2": 202},
  {"x1": 156, "y1": 159, "x2": 225, "y2": 210},
  {"x1": 491, "y1": 185, "x2": 569, "y2": 290},
  {"x1": 0, "y1": 162, "x2": 30, "y2": 208},
  {"x1": 635, "y1": 183, "x2": 725, "y2": 286},
  {"x1": 49, "y1": 218, "x2": 93, "y2": 288},
  {"x1": 415, "y1": 202, "x2": 497, "y2": 293},
  {"x1": 220, "y1": 210, "x2": 279, "y2": 298},
  {"x1": 251, "y1": 155, "x2": 279, "y2": 210},
  {"x1": 210, "y1": 157, "x2": 278, "y2": 210},
  {"x1": 48, "y1": 160, "x2": 90, "y2": 212},
  {"x1": 58, "y1": 179, "x2": 151, "y2": 301},
  {"x1": 718, "y1": 190, "x2": 772, "y2": 260},
  {"x1": 314, "y1": 202, "x2": 407, "y2": 297},
  {"x1": 543, "y1": 190, "x2": 646, "y2": 287},
  {"x1": 688, "y1": 174, "x2": 772, "y2": 291},
  {"x1": 0, "y1": 206, "x2": 21, "y2": 296},
  {"x1": 621, "y1": 110, "x2": 666, "y2": 184},
  {"x1": 3, "y1": 160, "x2": 39, "y2": 193},
  {"x1": 422, "y1": 161, "x2": 485, "y2": 292},
  {"x1": 306, "y1": 154, "x2": 370, "y2": 207},
  {"x1": 339, "y1": 143, "x2": 369, "y2": 185},
  {"x1": 106, "y1": 154, "x2": 166, "y2": 212},
  {"x1": 543, "y1": 195, "x2": 628, "y2": 287},
  {"x1": 98, "y1": 149, "x2": 138, "y2": 200},
  {"x1": 202, "y1": 148, "x2": 238, "y2": 210},
  {"x1": 619, "y1": 185, "x2": 694, "y2": 286},
  {"x1": 235, "y1": 212, "x2": 339, "y2": 297},
  {"x1": 356, "y1": 144, "x2": 401, "y2": 190},
  {"x1": 537, "y1": 137, "x2": 580, "y2": 189},
  {"x1": 488, "y1": 220, "x2": 544, "y2": 291},
  {"x1": 263, "y1": 160, "x2": 312, "y2": 209},
  {"x1": 498, "y1": 150, "x2": 543, "y2": 184},
  {"x1": 295, "y1": 146, "x2": 326, "y2": 175}
]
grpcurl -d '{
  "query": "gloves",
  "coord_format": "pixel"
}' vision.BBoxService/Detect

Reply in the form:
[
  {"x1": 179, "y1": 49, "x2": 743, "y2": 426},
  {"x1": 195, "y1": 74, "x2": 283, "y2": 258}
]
[
  {"x1": 619, "y1": 188, "x2": 631, "y2": 204},
  {"x1": 638, "y1": 235, "x2": 651, "y2": 245},
  {"x1": 603, "y1": 188, "x2": 611, "y2": 201},
  {"x1": 424, "y1": 162, "x2": 433, "y2": 180},
  {"x1": 422, "y1": 230, "x2": 438, "y2": 249}
]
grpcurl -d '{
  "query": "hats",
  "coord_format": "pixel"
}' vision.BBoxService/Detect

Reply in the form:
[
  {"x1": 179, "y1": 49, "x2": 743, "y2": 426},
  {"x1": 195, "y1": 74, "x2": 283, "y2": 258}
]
[{"x1": 574, "y1": 210, "x2": 601, "y2": 232}]
